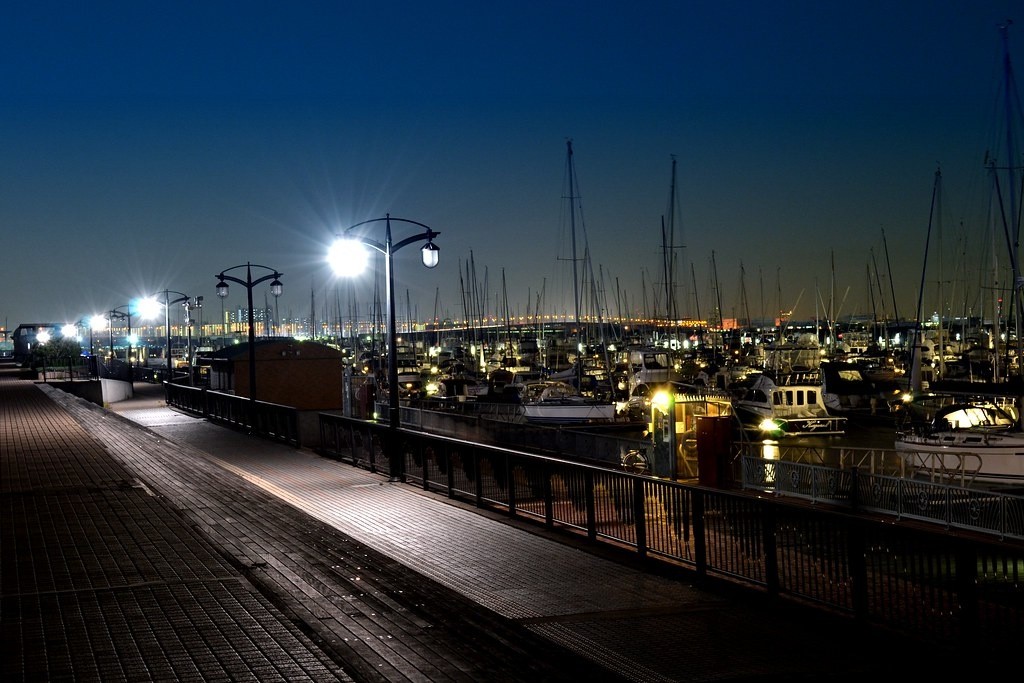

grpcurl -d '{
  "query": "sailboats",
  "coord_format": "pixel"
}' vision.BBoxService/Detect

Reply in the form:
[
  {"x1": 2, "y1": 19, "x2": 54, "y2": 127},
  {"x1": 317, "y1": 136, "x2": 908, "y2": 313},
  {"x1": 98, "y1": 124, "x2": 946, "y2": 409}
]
[{"x1": 310, "y1": 137, "x2": 1023, "y2": 485}]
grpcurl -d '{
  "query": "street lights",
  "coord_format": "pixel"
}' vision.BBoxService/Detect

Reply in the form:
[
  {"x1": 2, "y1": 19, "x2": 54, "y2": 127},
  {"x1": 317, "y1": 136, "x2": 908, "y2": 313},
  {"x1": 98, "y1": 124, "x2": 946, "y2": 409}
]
[
  {"x1": 214, "y1": 261, "x2": 284, "y2": 440},
  {"x1": 142, "y1": 288, "x2": 189, "y2": 382},
  {"x1": 334, "y1": 212, "x2": 443, "y2": 482},
  {"x1": 38, "y1": 303, "x2": 140, "y2": 383}
]
